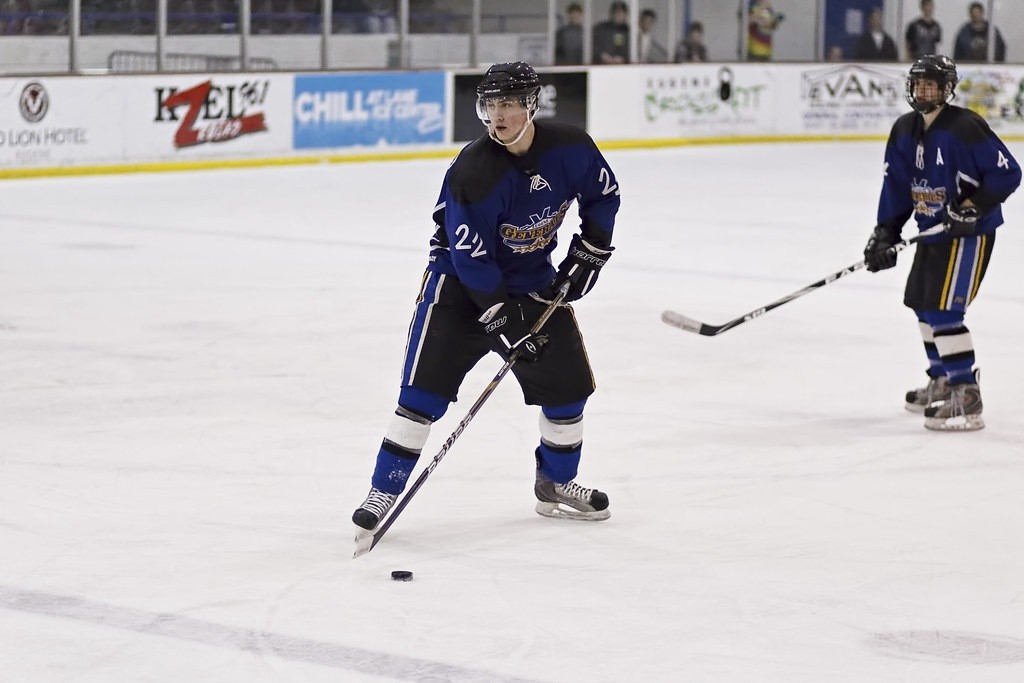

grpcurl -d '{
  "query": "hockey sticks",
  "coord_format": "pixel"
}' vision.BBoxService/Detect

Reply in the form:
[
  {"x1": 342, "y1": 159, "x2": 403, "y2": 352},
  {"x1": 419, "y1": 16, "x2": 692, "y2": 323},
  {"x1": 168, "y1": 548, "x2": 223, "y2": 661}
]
[
  {"x1": 349, "y1": 281, "x2": 572, "y2": 561},
  {"x1": 661, "y1": 222, "x2": 944, "y2": 336}
]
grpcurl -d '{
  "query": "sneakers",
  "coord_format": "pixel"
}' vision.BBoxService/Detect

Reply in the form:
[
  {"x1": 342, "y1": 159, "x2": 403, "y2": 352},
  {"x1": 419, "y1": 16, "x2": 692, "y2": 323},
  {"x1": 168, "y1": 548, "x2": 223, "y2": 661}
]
[
  {"x1": 352, "y1": 486, "x2": 397, "y2": 541},
  {"x1": 535, "y1": 446, "x2": 611, "y2": 520},
  {"x1": 905, "y1": 369, "x2": 947, "y2": 410},
  {"x1": 923, "y1": 368, "x2": 985, "y2": 430}
]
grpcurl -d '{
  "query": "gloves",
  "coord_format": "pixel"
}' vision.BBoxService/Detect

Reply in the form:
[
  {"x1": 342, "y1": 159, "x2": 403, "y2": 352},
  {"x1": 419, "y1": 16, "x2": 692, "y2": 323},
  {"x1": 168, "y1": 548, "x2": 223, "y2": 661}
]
[
  {"x1": 943, "y1": 200, "x2": 979, "y2": 236},
  {"x1": 476, "y1": 301, "x2": 550, "y2": 361},
  {"x1": 550, "y1": 233, "x2": 615, "y2": 302},
  {"x1": 866, "y1": 225, "x2": 900, "y2": 273}
]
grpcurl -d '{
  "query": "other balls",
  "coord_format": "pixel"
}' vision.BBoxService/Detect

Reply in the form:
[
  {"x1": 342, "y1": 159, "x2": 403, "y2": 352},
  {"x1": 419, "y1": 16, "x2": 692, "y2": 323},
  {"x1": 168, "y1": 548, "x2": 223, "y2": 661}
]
[{"x1": 391, "y1": 571, "x2": 414, "y2": 581}]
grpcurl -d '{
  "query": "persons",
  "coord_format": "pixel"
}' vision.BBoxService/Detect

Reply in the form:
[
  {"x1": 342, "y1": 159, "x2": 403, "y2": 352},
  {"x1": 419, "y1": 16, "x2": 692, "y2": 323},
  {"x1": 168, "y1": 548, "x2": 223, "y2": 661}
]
[
  {"x1": 954, "y1": 3, "x2": 1005, "y2": 63},
  {"x1": 636, "y1": 6, "x2": 666, "y2": 64},
  {"x1": 855, "y1": 8, "x2": 897, "y2": 62},
  {"x1": 736, "y1": 0, "x2": 786, "y2": 61},
  {"x1": 591, "y1": 1, "x2": 633, "y2": 67},
  {"x1": 350, "y1": 61, "x2": 621, "y2": 531},
  {"x1": 555, "y1": 3, "x2": 585, "y2": 68},
  {"x1": 672, "y1": 20, "x2": 708, "y2": 64},
  {"x1": 905, "y1": 0, "x2": 942, "y2": 61},
  {"x1": 864, "y1": 56, "x2": 1023, "y2": 418}
]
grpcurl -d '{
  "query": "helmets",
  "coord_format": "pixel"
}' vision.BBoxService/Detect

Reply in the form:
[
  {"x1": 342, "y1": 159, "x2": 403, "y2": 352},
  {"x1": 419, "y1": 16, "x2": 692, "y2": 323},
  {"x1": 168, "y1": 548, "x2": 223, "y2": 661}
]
[
  {"x1": 906, "y1": 55, "x2": 957, "y2": 113},
  {"x1": 476, "y1": 62, "x2": 542, "y2": 98}
]
